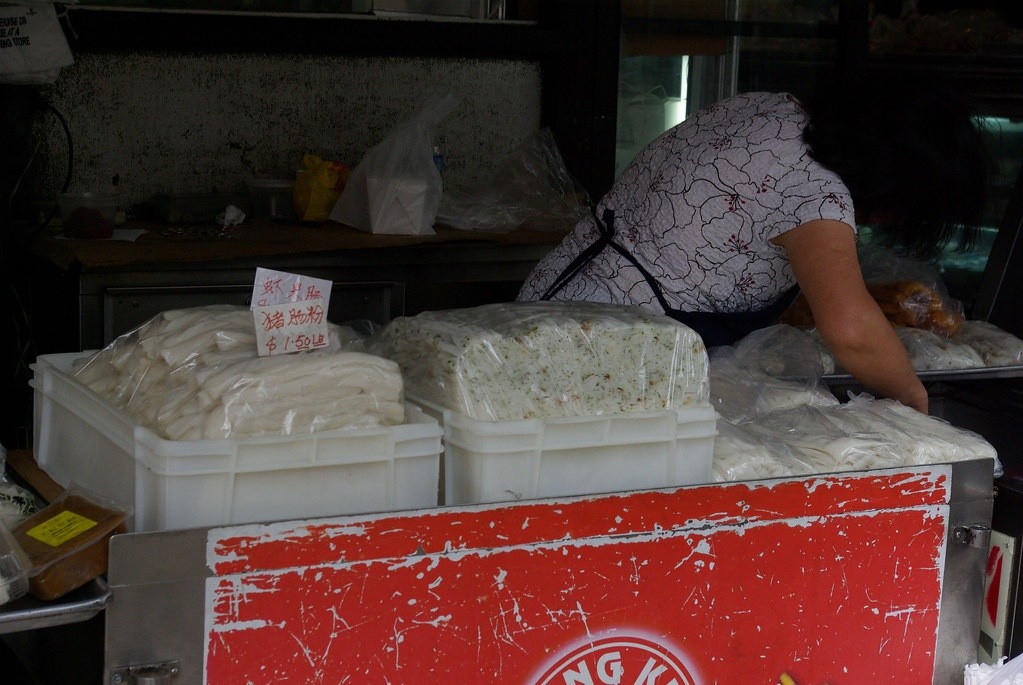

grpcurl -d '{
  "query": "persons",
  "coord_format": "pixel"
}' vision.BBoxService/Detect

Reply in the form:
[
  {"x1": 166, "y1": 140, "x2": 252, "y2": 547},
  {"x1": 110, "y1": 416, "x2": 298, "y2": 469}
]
[{"x1": 515, "y1": 48, "x2": 993, "y2": 412}]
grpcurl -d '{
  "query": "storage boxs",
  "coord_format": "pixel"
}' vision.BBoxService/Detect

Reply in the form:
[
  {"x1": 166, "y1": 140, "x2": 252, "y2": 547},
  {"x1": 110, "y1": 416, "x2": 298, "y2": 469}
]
[
  {"x1": 402, "y1": 389, "x2": 720, "y2": 507},
  {"x1": 26, "y1": 348, "x2": 446, "y2": 536}
]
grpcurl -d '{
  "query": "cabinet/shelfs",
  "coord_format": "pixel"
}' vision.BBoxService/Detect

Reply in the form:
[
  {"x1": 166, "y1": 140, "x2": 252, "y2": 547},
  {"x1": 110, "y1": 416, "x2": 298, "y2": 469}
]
[{"x1": 44, "y1": 225, "x2": 570, "y2": 351}]
